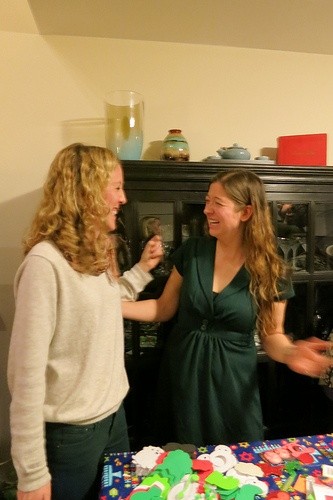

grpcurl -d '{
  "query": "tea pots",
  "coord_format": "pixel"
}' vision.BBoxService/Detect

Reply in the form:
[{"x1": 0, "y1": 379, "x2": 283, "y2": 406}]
[{"x1": 216, "y1": 143, "x2": 250, "y2": 159}]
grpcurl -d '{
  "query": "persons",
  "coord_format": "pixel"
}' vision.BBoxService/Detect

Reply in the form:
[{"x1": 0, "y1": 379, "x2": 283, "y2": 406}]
[
  {"x1": 6, "y1": 142, "x2": 164, "y2": 500},
  {"x1": 137, "y1": 203, "x2": 308, "y2": 355},
  {"x1": 122, "y1": 169, "x2": 333, "y2": 447}
]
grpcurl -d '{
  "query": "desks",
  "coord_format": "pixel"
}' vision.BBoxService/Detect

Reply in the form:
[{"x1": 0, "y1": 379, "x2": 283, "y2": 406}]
[{"x1": 99, "y1": 433, "x2": 333, "y2": 500}]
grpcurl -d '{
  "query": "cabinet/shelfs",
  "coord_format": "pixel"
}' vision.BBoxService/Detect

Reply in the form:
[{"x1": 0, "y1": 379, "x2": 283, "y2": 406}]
[{"x1": 119, "y1": 160, "x2": 333, "y2": 360}]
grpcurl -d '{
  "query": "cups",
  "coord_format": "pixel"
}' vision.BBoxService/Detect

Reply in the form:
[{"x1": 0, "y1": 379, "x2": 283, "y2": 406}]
[
  {"x1": 105, "y1": 90, "x2": 143, "y2": 160},
  {"x1": 208, "y1": 156, "x2": 222, "y2": 160},
  {"x1": 255, "y1": 156, "x2": 270, "y2": 160}
]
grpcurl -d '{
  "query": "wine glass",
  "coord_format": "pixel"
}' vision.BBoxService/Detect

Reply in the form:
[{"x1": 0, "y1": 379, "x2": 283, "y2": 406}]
[{"x1": 277, "y1": 236, "x2": 307, "y2": 272}]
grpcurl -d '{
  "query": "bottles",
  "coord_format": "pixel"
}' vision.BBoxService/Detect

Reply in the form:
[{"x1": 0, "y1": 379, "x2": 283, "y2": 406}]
[{"x1": 160, "y1": 130, "x2": 190, "y2": 162}]
